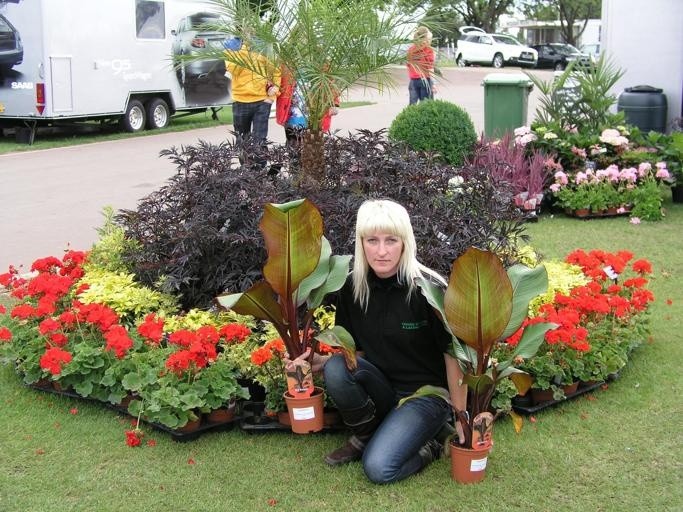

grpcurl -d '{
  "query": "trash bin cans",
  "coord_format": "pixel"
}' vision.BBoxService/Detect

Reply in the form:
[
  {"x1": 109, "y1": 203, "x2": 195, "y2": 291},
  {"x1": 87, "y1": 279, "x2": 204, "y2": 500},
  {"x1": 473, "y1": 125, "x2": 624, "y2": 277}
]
[{"x1": 481, "y1": 69, "x2": 534, "y2": 139}]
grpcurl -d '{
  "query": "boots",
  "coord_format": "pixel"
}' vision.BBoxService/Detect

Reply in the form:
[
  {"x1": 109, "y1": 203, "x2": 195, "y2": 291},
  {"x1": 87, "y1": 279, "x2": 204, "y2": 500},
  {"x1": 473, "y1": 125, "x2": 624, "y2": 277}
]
[
  {"x1": 416, "y1": 421, "x2": 459, "y2": 473},
  {"x1": 325, "y1": 396, "x2": 381, "y2": 466}
]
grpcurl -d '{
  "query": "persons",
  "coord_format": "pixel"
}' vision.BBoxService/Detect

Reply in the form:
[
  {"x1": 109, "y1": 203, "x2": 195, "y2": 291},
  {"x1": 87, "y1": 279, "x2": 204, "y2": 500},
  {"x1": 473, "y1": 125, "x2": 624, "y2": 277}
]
[
  {"x1": 221, "y1": 9, "x2": 282, "y2": 170},
  {"x1": 283, "y1": 196, "x2": 494, "y2": 485},
  {"x1": 263, "y1": 23, "x2": 341, "y2": 178},
  {"x1": 405, "y1": 25, "x2": 439, "y2": 105}
]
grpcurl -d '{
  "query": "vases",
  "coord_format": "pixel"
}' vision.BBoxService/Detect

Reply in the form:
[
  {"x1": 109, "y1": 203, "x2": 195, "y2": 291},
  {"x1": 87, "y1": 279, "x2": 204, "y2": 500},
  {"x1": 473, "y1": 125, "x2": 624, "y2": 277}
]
[{"x1": 531, "y1": 377, "x2": 595, "y2": 400}]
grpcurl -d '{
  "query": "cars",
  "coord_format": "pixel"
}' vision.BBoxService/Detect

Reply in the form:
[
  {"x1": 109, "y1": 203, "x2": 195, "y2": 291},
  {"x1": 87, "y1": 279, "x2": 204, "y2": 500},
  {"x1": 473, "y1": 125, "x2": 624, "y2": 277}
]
[
  {"x1": 455, "y1": 26, "x2": 601, "y2": 70},
  {"x1": 0, "y1": 14, "x2": 23, "y2": 69},
  {"x1": 171, "y1": 13, "x2": 239, "y2": 87}
]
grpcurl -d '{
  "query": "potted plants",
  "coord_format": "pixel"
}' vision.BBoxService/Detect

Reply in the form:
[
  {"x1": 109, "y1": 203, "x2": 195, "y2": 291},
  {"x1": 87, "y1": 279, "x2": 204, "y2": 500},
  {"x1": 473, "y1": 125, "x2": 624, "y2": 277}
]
[
  {"x1": 413, "y1": 245, "x2": 562, "y2": 485},
  {"x1": 217, "y1": 199, "x2": 357, "y2": 435}
]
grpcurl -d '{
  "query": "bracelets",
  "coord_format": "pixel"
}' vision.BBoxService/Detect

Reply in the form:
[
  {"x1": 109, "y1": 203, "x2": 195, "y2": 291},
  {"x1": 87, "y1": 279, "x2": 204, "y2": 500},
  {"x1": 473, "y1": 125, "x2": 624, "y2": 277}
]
[{"x1": 450, "y1": 408, "x2": 469, "y2": 424}]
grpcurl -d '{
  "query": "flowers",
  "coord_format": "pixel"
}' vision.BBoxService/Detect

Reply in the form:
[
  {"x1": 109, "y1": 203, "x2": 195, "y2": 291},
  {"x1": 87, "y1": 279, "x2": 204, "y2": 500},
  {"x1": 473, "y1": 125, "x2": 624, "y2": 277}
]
[
  {"x1": 514, "y1": 125, "x2": 669, "y2": 224},
  {"x1": 506, "y1": 248, "x2": 656, "y2": 390},
  {"x1": 0, "y1": 250, "x2": 313, "y2": 449}
]
